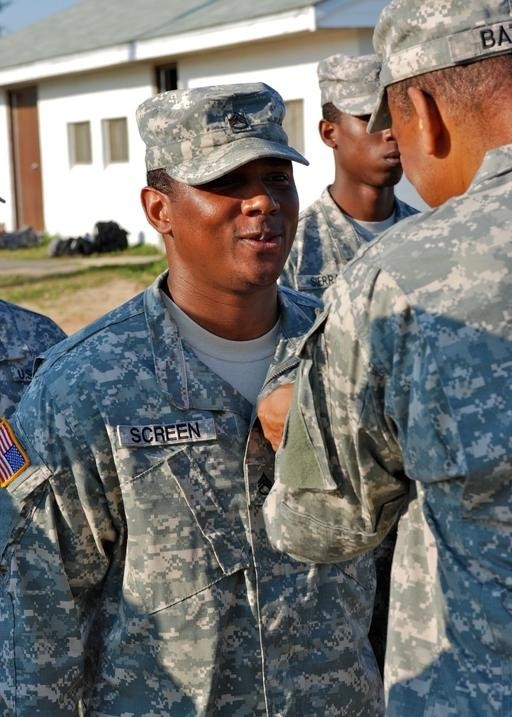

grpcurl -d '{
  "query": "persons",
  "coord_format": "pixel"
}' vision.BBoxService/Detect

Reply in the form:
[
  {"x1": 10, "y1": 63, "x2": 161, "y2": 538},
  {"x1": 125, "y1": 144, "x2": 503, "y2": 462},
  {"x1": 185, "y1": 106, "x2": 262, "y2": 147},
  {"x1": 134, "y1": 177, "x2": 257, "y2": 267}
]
[
  {"x1": 1, "y1": 300, "x2": 72, "y2": 422},
  {"x1": 1, "y1": 78, "x2": 385, "y2": 715},
  {"x1": 272, "y1": 51, "x2": 421, "y2": 300},
  {"x1": 250, "y1": 1, "x2": 510, "y2": 717}
]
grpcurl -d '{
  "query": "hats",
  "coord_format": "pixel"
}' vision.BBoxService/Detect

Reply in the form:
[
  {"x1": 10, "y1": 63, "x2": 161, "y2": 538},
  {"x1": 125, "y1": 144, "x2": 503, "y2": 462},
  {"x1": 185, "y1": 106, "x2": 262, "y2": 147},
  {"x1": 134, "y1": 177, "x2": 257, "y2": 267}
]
[
  {"x1": 317, "y1": 54, "x2": 383, "y2": 119},
  {"x1": 366, "y1": 1, "x2": 512, "y2": 132},
  {"x1": 135, "y1": 82, "x2": 306, "y2": 187}
]
